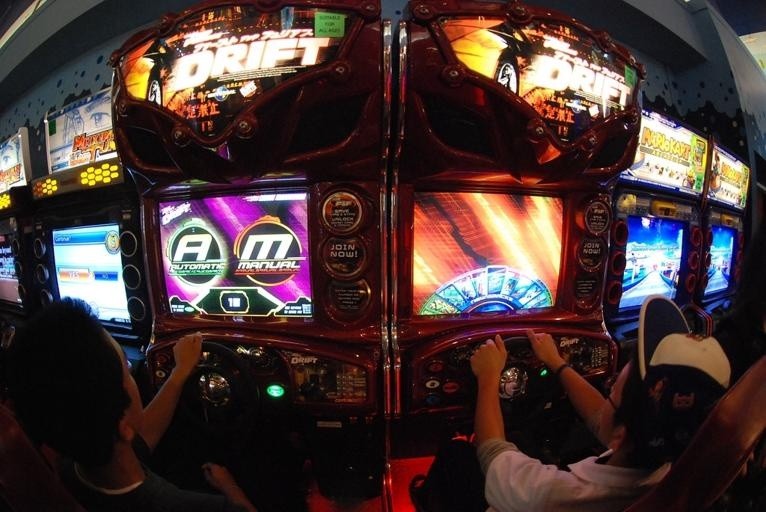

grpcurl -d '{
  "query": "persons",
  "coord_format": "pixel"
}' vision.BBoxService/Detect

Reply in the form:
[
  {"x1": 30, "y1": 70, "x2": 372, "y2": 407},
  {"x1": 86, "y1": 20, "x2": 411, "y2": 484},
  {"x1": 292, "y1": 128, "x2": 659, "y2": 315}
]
[
  {"x1": 469, "y1": 296, "x2": 732, "y2": 512},
  {"x1": 0, "y1": 297, "x2": 257, "y2": 512}
]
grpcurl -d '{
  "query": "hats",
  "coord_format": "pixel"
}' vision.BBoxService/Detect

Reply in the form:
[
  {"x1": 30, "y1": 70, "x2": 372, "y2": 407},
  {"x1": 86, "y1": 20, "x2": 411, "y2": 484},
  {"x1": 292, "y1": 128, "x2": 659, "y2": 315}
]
[{"x1": 637, "y1": 293, "x2": 732, "y2": 420}]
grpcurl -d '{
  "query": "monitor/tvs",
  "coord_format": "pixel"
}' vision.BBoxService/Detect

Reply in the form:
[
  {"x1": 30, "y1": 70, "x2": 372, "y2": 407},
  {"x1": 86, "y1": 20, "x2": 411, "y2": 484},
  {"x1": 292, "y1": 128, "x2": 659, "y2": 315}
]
[
  {"x1": 0, "y1": 216, "x2": 32, "y2": 319},
  {"x1": 30, "y1": 188, "x2": 153, "y2": 349},
  {"x1": 401, "y1": 181, "x2": 569, "y2": 316},
  {"x1": 155, "y1": 185, "x2": 313, "y2": 320},
  {"x1": 693, "y1": 205, "x2": 746, "y2": 307},
  {"x1": 604, "y1": 189, "x2": 702, "y2": 326}
]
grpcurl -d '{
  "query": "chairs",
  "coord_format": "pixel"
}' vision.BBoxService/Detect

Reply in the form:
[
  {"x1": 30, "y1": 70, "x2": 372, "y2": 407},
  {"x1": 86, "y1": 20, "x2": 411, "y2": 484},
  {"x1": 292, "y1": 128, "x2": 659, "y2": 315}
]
[{"x1": 620, "y1": 356, "x2": 766, "y2": 510}]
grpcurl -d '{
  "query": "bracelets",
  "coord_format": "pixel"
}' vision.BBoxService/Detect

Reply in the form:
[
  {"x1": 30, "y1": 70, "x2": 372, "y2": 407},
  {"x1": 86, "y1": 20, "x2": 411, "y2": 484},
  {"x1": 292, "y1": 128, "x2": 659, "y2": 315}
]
[{"x1": 554, "y1": 363, "x2": 572, "y2": 380}]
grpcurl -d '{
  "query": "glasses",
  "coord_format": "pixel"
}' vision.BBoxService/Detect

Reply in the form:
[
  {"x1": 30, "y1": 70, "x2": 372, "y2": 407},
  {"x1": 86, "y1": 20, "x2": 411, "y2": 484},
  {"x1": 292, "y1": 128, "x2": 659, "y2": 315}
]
[{"x1": 602, "y1": 375, "x2": 618, "y2": 411}]
